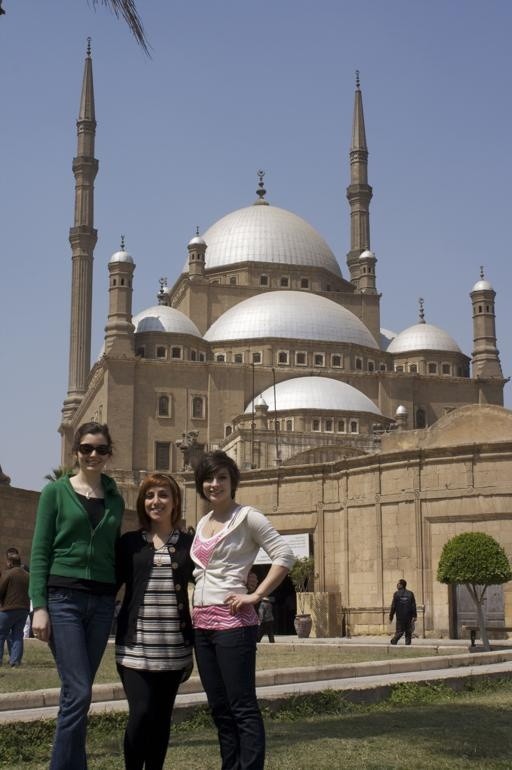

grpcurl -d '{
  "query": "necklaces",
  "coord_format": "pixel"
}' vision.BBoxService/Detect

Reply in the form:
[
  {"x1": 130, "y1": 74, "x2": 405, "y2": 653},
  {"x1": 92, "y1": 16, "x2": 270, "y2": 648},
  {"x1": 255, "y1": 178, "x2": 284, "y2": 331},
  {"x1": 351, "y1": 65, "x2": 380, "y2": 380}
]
[
  {"x1": 152, "y1": 529, "x2": 174, "y2": 567},
  {"x1": 208, "y1": 507, "x2": 232, "y2": 536},
  {"x1": 78, "y1": 478, "x2": 101, "y2": 500}
]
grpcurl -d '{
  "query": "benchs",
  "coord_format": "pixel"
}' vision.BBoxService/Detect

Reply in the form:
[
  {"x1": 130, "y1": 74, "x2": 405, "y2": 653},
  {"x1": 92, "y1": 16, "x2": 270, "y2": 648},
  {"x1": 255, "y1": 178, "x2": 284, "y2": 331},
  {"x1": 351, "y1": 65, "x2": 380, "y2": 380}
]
[{"x1": 463, "y1": 623, "x2": 512, "y2": 646}]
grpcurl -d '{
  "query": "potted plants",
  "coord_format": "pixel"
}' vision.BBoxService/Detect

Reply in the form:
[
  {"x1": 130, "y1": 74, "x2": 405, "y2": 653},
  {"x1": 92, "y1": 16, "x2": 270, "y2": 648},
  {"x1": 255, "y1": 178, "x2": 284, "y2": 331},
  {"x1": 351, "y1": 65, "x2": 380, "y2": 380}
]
[{"x1": 288, "y1": 557, "x2": 316, "y2": 637}]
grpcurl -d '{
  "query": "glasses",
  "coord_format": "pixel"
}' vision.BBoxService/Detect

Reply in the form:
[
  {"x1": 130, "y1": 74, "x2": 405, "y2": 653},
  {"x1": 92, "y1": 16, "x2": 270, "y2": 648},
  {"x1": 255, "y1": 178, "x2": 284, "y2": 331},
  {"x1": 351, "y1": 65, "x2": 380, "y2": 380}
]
[{"x1": 79, "y1": 442, "x2": 108, "y2": 456}]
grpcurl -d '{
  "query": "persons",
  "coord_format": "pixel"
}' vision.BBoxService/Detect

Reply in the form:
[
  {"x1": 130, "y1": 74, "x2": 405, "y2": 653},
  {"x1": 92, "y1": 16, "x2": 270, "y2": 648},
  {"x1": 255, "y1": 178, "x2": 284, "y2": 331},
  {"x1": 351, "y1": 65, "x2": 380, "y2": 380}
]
[
  {"x1": 0, "y1": 553, "x2": 33, "y2": 668},
  {"x1": 115, "y1": 473, "x2": 258, "y2": 770},
  {"x1": 188, "y1": 449, "x2": 297, "y2": 770},
  {"x1": 111, "y1": 600, "x2": 121, "y2": 635},
  {"x1": 29, "y1": 422, "x2": 125, "y2": 770},
  {"x1": 257, "y1": 596, "x2": 275, "y2": 643},
  {"x1": 388, "y1": 579, "x2": 417, "y2": 645}
]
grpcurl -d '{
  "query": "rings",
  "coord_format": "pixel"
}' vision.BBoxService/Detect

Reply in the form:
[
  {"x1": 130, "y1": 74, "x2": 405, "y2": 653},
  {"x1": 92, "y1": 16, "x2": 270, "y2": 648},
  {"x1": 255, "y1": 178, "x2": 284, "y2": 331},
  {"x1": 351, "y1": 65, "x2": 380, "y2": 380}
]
[
  {"x1": 34, "y1": 633, "x2": 37, "y2": 637},
  {"x1": 250, "y1": 585, "x2": 253, "y2": 589}
]
